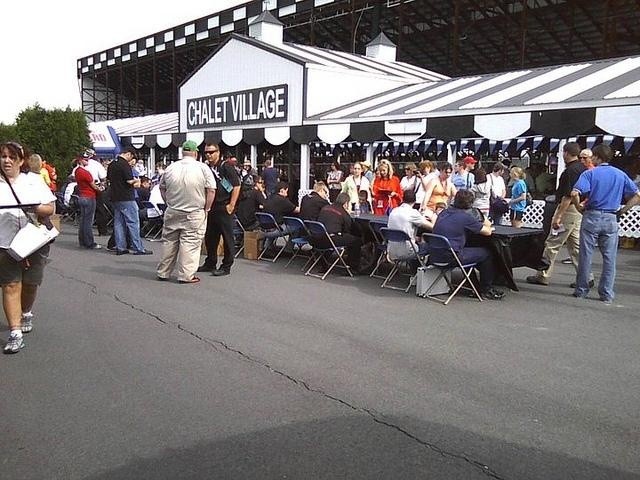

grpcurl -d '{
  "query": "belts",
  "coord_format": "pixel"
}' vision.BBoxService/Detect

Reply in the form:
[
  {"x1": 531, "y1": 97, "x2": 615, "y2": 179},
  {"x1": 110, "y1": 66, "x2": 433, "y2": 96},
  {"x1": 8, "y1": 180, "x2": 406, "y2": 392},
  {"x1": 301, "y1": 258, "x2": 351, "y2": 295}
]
[{"x1": 594, "y1": 209, "x2": 617, "y2": 214}]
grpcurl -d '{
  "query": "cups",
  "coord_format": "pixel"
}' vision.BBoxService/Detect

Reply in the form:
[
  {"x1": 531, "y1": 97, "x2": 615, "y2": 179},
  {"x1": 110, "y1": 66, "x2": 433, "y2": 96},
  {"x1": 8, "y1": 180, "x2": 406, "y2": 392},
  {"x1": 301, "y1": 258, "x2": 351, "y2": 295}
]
[{"x1": 354, "y1": 208, "x2": 363, "y2": 218}]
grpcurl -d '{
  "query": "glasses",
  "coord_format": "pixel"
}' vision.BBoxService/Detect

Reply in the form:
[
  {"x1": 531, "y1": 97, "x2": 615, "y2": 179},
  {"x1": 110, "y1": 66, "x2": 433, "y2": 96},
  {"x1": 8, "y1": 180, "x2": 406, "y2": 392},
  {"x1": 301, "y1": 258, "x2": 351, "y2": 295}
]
[
  {"x1": 404, "y1": 168, "x2": 411, "y2": 170},
  {"x1": 204, "y1": 151, "x2": 218, "y2": 154},
  {"x1": 579, "y1": 157, "x2": 588, "y2": 159},
  {"x1": 355, "y1": 168, "x2": 361, "y2": 169},
  {"x1": 444, "y1": 171, "x2": 451, "y2": 173}
]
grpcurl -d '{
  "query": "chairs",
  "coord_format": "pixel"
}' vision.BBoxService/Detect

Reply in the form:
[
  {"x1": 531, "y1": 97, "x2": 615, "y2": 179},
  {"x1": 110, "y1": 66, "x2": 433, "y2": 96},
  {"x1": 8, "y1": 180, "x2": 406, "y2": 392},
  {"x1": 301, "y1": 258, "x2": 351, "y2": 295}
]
[
  {"x1": 103, "y1": 203, "x2": 114, "y2": 226},
  {"x1": 282, "y1": 216, "x2": 325, "y2": 271},
  {"x1": 304, "y1": 219, "x2": 353, "y2": 280},
  {"x1": 233, "y1": 213, "x2": 246, "y2": 258},
  {"x1": 255, "y1": 212, "x2": 301, "y2": 263},
  {"x1": 51, "y1": 190, "x2": 80, "y2": 226},
  {"x1": 379, "y1": 227, "x2": 426, "y2": 293},
  {"x1": 141, "y1": 201, "x2": 163, "y2": 237},
  {"x1": 369, "y1": 221, "x2": 402, "y2": 282},
  {"x1": 153, "y1": 203, "x2": 167, "y2": 239},
  {"x1": 421, "y1": 232, "x2": 483, "y2": 305}
]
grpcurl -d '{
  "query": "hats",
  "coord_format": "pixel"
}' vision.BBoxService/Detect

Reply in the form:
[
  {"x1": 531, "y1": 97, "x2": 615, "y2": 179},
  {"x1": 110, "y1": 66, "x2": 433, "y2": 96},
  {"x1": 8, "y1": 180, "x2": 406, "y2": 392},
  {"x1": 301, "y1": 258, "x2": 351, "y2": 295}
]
[
  {"x1": 182, "y1": 141, "x2": 199, "y2": 151},
  {"x1": 463, "y1": 156, "x2": 478, "y2": 164},
  {"x1": 493, "y1": 161, "x2": 507, "y2": 171},
  {"x1": 79, "y1": 150, "x2": 89, "y2": 160}
]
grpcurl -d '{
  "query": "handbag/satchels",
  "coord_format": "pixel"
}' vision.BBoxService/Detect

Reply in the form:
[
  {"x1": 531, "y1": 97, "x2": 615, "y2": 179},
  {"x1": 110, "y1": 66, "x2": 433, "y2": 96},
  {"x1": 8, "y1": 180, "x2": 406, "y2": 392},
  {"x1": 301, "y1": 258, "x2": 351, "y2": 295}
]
[
  {"x1": 6, "y1": 216, "x2": 60, "y2": 261},
  {"x1": 489, "y1": 190, "x2": 509, "y2": 222},
  {"x1": 524, "y1": 191, "x2": 533, "y2": 208}
]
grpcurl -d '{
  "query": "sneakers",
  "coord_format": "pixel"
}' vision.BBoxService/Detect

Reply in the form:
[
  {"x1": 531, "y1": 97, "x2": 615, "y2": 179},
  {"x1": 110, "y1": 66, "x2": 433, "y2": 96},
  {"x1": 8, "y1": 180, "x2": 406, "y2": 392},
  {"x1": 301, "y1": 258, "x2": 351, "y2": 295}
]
[
  {"x1": 570, "y1": 280, "x2": 594, "y2": 288},
  {"x1": 178, "y1": 276, "x2": 200, "y2": 283},
  {"x1": 482, "y1": 289, "x2": 505, "y2": 299},
  {"x1": 20, "y1": 316, "x2": 33, "y2": 333},
  {"x1": 134, "y1": 249, "x2": 153, "y2": 255},
  {"x1": 346, "y1": 269, "x2": 362, "y2": 276},
  {"x1": 469, "y1": 289, "x2": 481, "y2": 297},
  {"x1": 600, "y1": 297, "x2": 613, "y2": 304},
  {"x1": 4, "y1": 334, "x2": 25, "y2": 353},
  {"x1": 527, "y1": 276, "x2": 550, "y2": 285},
  {"x1": 117, "y1": 250, "x2": 129, "y2": 255},
  {"x1": 561, "y1": 258, "x2": 572, "y2": 263},
  {"x1": 157, "y1": 276, "x2": 170, "y2": 281},
  {"x1": 88, "y1": 243, "x2": 101, "y2": 249},
  {"x1": 572, "y1": 291, "x2": 588, "y2": 298}
]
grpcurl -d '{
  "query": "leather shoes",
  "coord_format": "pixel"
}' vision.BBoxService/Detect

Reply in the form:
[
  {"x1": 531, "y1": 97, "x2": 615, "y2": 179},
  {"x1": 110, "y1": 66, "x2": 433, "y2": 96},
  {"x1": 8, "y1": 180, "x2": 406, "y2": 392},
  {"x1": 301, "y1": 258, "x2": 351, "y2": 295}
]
[
  {"x1": 197, "y1": 263, "x2": 216, "y2": 272},
  {"x1": 213, "y1": 269, "x2": 229, "y2": 276}
]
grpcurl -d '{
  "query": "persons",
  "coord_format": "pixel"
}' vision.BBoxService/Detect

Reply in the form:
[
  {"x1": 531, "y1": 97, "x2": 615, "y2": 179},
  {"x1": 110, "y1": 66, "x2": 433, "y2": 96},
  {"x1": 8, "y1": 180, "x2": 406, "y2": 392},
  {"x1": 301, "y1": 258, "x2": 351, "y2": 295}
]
[
  {"x1": 0, "y1": 141, "x2": 57, "y2": 353},
  {"x1": 25, "y1": 142, "x2": 640, "y2": 303}
]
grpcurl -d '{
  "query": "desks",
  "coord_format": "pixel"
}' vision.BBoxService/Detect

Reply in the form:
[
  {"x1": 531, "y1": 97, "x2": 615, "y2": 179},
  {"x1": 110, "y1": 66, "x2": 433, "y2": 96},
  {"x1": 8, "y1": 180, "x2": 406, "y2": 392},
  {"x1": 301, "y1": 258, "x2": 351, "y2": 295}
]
[{"x1": 351, "y1": 213, "x2": 544, "y2": 291}]
